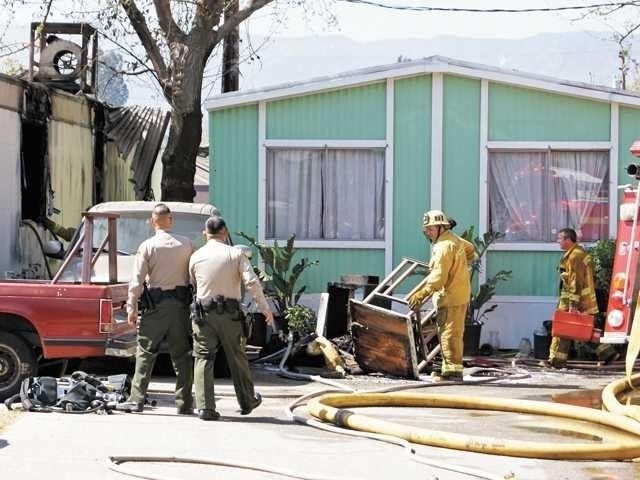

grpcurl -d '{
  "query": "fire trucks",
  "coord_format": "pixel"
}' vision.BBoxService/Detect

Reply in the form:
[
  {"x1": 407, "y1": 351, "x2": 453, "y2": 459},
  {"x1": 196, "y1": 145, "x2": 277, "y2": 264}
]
[
  {"x1": 498, "y1": 166, "x2": 608, "y2": 244},
  {"x1": 600, "y1": 140, "x2": 640, "y2": 359}
]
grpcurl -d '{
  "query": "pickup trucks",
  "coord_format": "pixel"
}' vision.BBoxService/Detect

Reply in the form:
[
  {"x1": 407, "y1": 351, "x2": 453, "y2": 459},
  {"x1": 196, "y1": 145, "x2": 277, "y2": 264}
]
[{"x1": 0, "y1": 278, "x2": 146, "y2": 404}]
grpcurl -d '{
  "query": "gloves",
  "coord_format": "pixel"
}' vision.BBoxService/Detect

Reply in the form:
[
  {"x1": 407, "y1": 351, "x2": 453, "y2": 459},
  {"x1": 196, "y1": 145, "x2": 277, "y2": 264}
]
[{"x1": 408, "y1": 287, "x2": 428, "y2": 310}]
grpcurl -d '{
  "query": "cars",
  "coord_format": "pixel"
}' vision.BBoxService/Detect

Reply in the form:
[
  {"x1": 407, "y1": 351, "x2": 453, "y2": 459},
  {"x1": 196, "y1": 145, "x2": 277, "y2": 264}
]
[
  {"x1": 267, "y1": 182, "x2": 382, "y2": 242},
  {"x1": 64, "y1": 199, "x2": 246, "y2": 379}
]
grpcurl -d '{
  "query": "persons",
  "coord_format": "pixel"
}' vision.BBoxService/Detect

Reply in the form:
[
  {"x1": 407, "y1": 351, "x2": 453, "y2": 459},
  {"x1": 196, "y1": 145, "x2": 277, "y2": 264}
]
[
  {"x1": 539, "y1": 227, "x2": 620, "y2": 370},
  {"x1": 188, "y1": 215, "x2": 273, "y2": 421},
  {"x1": 407, "y1": 210, "x2": 476, "y2": 382},
  {"x1": 125, "y1": 203, "x2": 198, "y2": 415}
]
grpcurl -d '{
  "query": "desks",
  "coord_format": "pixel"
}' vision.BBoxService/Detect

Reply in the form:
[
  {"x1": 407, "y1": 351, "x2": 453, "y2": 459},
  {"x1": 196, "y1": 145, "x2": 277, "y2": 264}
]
[{"x1": 344, "y1": 254, "x2": 443, "y2": 381}]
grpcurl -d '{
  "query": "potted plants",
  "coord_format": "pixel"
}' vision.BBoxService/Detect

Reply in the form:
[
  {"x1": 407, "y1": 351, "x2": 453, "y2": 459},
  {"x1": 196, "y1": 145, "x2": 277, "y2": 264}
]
[
  {"x1": 458, "y1": 225, "x2": 513, "y2": 357},
  {"x1": 231, "y1": 227, "x2": 321, "y2": 356}
]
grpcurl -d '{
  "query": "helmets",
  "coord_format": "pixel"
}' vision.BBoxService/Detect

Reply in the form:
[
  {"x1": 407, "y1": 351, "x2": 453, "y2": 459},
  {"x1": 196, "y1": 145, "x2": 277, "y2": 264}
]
[{"x1": 423, "y1": 209, "x2": 450, "y2": 228}]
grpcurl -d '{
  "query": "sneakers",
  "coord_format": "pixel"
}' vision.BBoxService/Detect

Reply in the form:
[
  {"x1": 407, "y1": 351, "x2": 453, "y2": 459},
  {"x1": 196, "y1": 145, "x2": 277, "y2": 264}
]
[
  {"x1": 177, "y1": 407, "x2": 195, "y2": 414},
  {"x1": 539, "y1": 357, "x2": 568, "y2": 369},
  {"x1": 199, "y1": 408, "x2": 220, "y2": 419},
  {"x1": 598, "y1": 352, "x2": 620, "y2": 366},
  {"x1": 431, "y1": 370, "x2": 462, "y2": 381},
  {"x1": 239, "y1": 392, "x2": 261, "y2": 414},
  {"x1": 115, "y1": 402, "x2": 143, "y2": 412}
]
